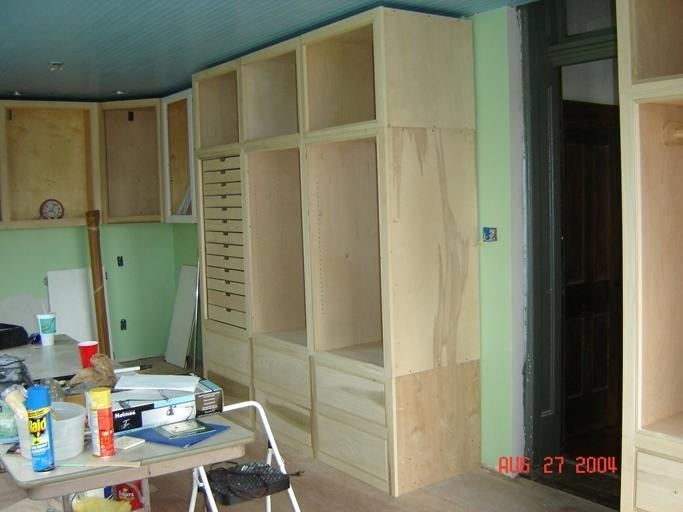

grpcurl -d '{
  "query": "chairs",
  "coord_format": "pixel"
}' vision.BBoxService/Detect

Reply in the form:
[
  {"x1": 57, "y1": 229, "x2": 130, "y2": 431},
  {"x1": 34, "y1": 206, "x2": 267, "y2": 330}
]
[{"x1": 187, "y1": 400, "x2": 302, "y2": 512}]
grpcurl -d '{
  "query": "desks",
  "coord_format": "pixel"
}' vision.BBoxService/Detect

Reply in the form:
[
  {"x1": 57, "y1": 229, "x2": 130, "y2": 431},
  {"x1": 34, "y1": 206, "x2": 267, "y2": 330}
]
[{"x1": 0, "y1": 414, "x2": 257, "y2": 512}]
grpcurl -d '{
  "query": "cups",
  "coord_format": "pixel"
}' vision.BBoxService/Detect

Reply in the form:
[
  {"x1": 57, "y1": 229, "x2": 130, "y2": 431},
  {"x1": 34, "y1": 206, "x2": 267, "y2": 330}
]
[
  {"x1": 36, "y1": 313, "x2": 58, "y2": 346},
  {"x1": 77, "y1": 339, "x2": 99, "y2": 369}
]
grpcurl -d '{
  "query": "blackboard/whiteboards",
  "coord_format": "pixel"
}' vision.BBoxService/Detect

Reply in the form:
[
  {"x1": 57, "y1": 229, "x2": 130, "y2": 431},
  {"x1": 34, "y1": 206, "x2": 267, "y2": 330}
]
[
  {"x1": 164, "y1": 264, "x2": 198, "y2": 369},
  {"x1": 47, "y1": 267, "x2": 114, "y2": 362}
]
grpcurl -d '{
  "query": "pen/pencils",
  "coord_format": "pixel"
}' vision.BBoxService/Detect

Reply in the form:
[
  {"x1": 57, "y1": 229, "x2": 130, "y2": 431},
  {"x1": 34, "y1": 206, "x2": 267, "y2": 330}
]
[{"x1": 113, "y1": 365, "x2": 153, "y2": 374}]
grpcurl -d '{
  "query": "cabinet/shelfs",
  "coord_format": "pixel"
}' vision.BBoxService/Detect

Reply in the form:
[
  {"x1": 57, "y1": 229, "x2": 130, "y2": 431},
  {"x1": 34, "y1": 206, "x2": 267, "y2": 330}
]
[{"x1": 613, "y1": 1, "x2": 683, "y2": 460}]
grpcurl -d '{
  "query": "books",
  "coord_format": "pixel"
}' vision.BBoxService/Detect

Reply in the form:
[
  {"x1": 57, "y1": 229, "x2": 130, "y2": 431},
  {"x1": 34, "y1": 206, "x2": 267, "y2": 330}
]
[{"x1": 107, "y1": 369, "x2": 199, "y2": 394}]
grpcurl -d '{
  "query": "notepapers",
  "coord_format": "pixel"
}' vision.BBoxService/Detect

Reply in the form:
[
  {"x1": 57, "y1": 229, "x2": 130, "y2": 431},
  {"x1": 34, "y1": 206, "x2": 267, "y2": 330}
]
[{"x1": 114, "y1": 436, "x2": 145, "y2": 450}]
